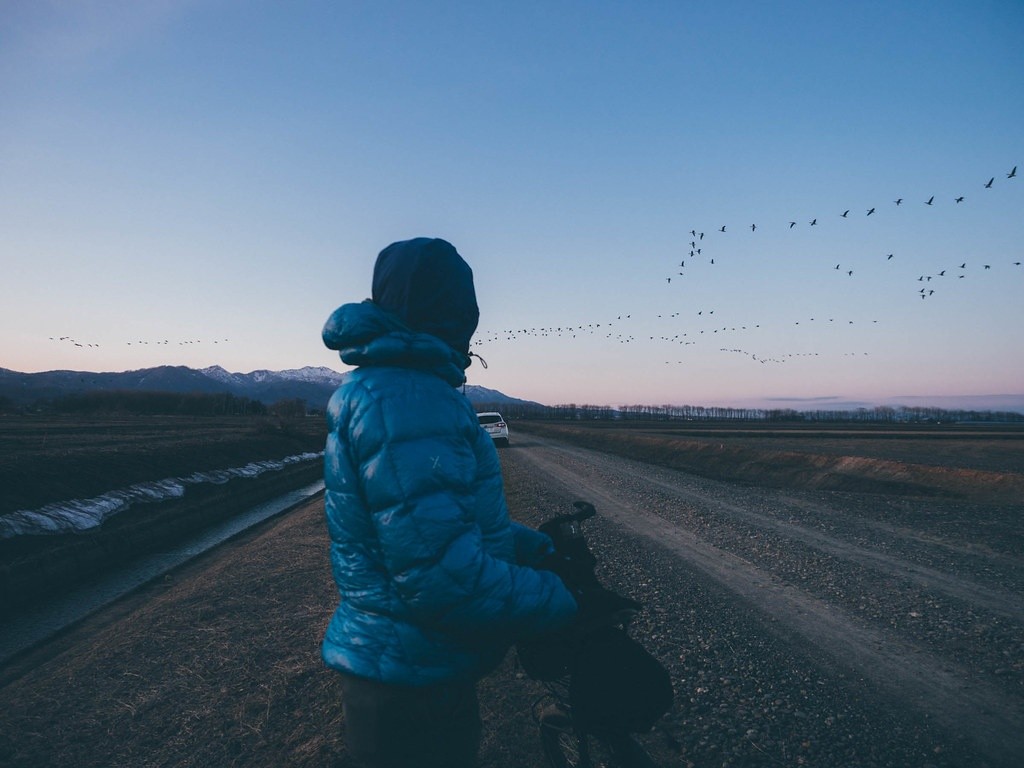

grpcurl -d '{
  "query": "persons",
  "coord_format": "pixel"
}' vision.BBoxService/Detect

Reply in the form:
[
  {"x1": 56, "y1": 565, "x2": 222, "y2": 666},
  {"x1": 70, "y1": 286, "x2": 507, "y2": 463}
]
[{"x1": 320, "y1": 238, "x2": 579, "y2": 768}]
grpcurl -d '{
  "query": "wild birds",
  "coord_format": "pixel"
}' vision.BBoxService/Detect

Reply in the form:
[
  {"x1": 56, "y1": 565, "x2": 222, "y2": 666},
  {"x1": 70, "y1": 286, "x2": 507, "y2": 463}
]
[
  {"x1": 924, "y1": 176, "x2": 994, "y2": 205},
  {"x1": 469, "y1": 313, "x2": 634, "y2": 344},
  {"x1": 720, "y1": 348, "x2": 818, "y2": 363},
  {"x1": 657, "y1": 309, "x2": 714, "y2": 318},
  {"x1": 839, "y1": 198, "x2": 903, "y2": 217},
  {"x1": 887, "y1": 254, "x2": 893, "y2": 260},
  {"x1": 50, "y1": 336, "x2": 99, "y2": 348},
  {"x1": 915, "y1": 262, "x2": 1020, "y2": 300},
  {"x1": 650, "y1": 332, "x2": 695, "y2": 364},
  {"x1": 1006, "y1": 166, "x2": 1017, "y2": 178},
  {"x1": 789, "y1": 222, "x2": 796, "y2": 228},
  {"x1": 718, "y1": 225, "x2": 726, "y2": 232},
  {"x1": 701, "y1": 325, "x2": 759, "y2": 334},
  {"x1": 750, "y1": 224, "x2": 756, "y2": 231},
  {"x1": 796, "y1": 318, "x2": 877, "y2": 325},
  {"x1": 809, "y1": 218, "x2": 818, "y2": 226},
  {"x1": 666, "y1": 230, "x2": 714, "y2": 284},
  {"x1": 127, "y1": 338, "x2": 229, "y2": 345},
  {"x1": 832, "y1": 263, "x2": 853, "y2": 275},
  {"x1": 844, "y1": 352, "x2": 867, "y2": 356}
]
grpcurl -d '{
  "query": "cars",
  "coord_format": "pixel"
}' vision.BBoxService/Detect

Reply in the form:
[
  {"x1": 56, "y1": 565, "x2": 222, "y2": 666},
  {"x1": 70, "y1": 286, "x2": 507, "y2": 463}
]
[{"x1": 475, "y1": 411, "x2": 511, "y2": 448}]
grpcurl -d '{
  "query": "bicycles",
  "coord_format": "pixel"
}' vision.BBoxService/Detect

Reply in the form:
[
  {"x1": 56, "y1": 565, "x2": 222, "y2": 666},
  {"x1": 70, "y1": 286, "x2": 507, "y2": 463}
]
[{"x1": 510, "y1": 498, "x2": 670, "y2": 768}]
